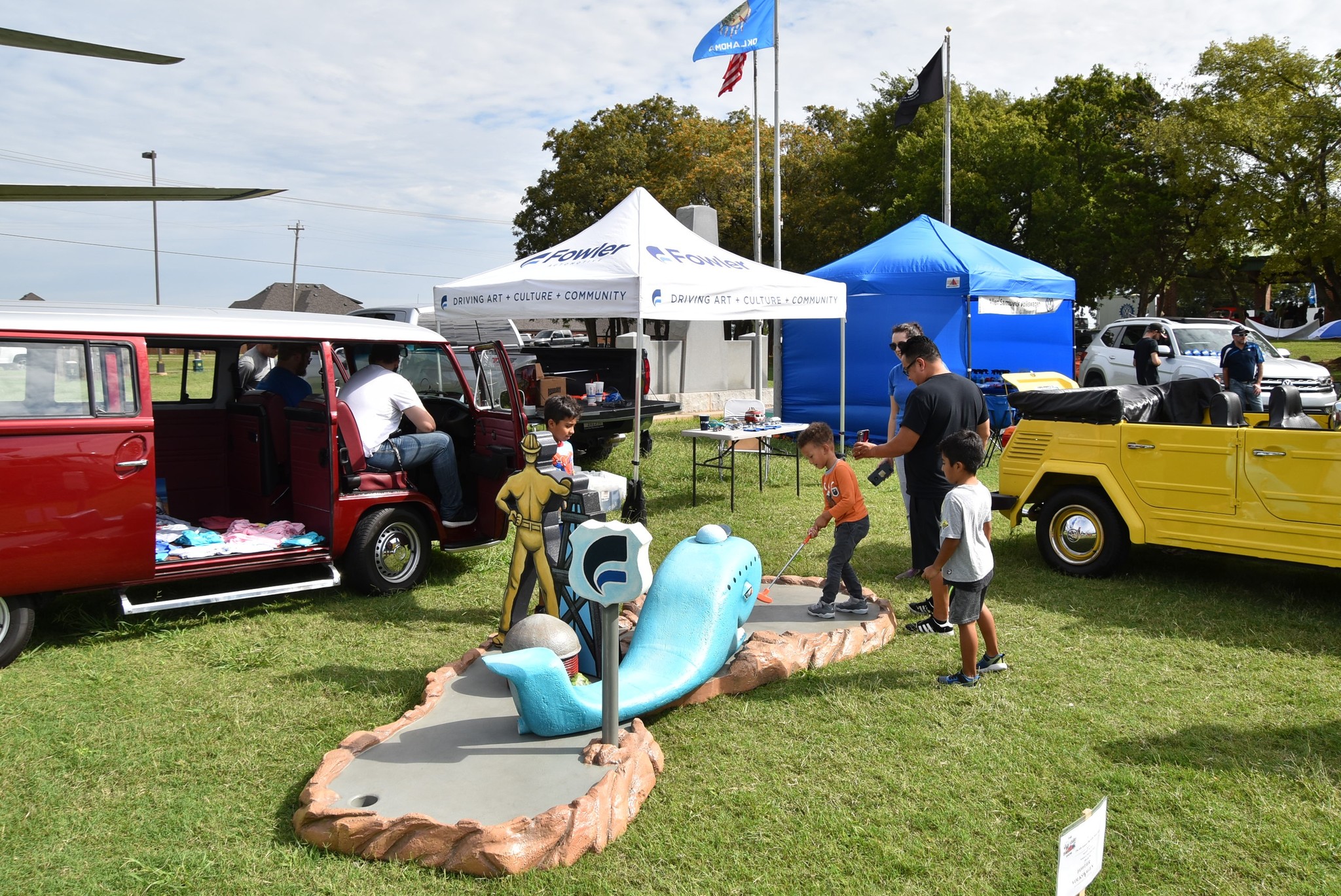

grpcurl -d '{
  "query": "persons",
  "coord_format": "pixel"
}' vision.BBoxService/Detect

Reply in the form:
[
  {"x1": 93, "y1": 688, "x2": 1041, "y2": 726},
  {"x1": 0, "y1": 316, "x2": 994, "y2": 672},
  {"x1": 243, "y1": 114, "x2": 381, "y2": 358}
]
[
  {"x1": 852, "y1": 335, "x2": 990, "y2": 636},
  {"x1": 336, "y1": 343, "x2": 478, "y2": 529},
  {"x1": 1220, "y1": 326, "x2": 1265, "y2": 412},
  {"x1": 238, "y1": 343, "x2": 279, "y2": 391},
  {"x1": 921, "y1": 428, "x2": 1008, "y2": 689},
  {"x1": 255, "y1": 342, "x2": 313, "y2": 408},
  {"x1": 876, "y1": 322, "x2": 924, "y2": 580},
  {"x1": 1258, "y1": 299, "x2": 1323, "y2": 328},
  {"x1": 1133, "y1": 323, "x2": 1164, "y2": 386},
  {"x1": 193, "y1": 349, "x2": 205, "y2": 359},
  {"x1": 798, "y1": 422, "x2": 870, "y2": 619},
  {"x1": 534, "y1": 395, "x2": 584, "y2": 614}
]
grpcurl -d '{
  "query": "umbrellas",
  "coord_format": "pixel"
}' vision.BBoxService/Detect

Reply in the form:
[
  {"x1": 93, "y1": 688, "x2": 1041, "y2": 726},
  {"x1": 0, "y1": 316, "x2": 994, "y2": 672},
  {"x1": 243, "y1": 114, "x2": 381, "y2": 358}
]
[{"x1": 1307, "y1": 319, "x2": 1341, "y2": 339}]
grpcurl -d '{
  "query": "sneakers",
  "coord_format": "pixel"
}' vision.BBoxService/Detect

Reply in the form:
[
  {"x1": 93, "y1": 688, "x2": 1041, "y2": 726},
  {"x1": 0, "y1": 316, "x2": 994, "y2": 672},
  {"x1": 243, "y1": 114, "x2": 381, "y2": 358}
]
[
  {"x1": 904, "y1": 599, "x2": 955, "y2": 636},
  {"x1": 441, "y1": 506, "x2": 479, "y2": 528},
  {"x1": 936, "y1": 652, "x2": 1007, "y2": 688},
  {"x1": 895, "y1": 568, "x2": 919, "y2": 580},
  {"x1": 807, "y1": 594, "x2": 868, "y2": 618}
]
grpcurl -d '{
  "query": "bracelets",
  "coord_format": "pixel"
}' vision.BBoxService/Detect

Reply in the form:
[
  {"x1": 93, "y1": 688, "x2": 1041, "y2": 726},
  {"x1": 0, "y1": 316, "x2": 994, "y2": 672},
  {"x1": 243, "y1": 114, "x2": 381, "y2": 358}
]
[{"x1": 1225, "y1": 387, "x2": 1229, "y2": 390}]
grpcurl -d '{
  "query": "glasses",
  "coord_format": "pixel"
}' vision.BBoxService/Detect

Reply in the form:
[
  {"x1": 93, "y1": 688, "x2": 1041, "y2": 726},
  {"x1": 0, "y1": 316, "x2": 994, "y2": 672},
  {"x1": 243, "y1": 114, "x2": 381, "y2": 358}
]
[
  {"x1": 272, "y1": 344, "x2": 279, "y2": 349},
  {"x1": 300, "y1": 353, "x2": 312, "y2": 362},
  {"x1": 903, "y1": 354, "x2": 923, "y2": 376},
  {"x1": 889, "y1": 342, "x2": 907, "y2": 351},
  {"x1": 1234, "y1": 334, "x2": 1248, "y2": 337}
]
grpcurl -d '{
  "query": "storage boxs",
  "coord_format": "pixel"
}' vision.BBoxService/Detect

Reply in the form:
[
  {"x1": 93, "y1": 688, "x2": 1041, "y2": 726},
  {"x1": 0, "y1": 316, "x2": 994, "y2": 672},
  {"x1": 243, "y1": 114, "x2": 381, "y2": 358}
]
[
  {"x1": 536, "y1": 363, "x2": 576, "y2": 406},
  {"x1": 577, "y1": 470, "x2": 626, "y2": 512}
]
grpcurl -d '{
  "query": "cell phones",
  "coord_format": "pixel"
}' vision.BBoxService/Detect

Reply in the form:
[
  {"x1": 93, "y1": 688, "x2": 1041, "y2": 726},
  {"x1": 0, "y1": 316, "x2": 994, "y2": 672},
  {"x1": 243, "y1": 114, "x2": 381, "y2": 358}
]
[
  {"x1": 857, "y1": 429, "x2": 870, "y2": 442},
  {"x1": 867, "y1": 461, "x2": 894, "y2": 486}
]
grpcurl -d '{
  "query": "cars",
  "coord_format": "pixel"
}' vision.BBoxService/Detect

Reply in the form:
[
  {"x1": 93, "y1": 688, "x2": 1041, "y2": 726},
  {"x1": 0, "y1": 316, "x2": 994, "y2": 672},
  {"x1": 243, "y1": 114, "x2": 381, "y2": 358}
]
[
  {"x1": 987, "y1": 371, "x2": 1341, "y2": 578},
  {"x1": 519, "y1": 334, "x2": 534, "y2": 347},
  {"x1": 1078, "y1": 316, "x2": 1337, "y2": 414},
  {"x1": 0, "y1": 347, "x2": 27, "y2": 371}
]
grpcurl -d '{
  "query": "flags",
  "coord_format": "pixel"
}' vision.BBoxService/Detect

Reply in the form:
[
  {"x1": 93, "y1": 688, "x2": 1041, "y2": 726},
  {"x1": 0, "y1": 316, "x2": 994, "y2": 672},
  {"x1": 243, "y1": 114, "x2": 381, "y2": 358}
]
[
  {"x1": 1308, "y1": 282, "x2": 1315, "y2": 304},
  {"x1": 718, "y1": 52, "x2": 747, "y2": 97},
  {"x1": 894, "y1": 40, "x2": 946, "y2": 130},
  {"x1": 693, "y1": 0, "x2": 774, "y2": 62}
]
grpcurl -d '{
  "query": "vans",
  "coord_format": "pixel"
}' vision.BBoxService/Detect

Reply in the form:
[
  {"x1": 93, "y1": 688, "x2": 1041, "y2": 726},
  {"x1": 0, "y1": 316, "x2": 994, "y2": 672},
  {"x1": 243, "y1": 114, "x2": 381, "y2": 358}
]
[{"x1": 0, "y1": 301, "x2": 529, "y2": 676}]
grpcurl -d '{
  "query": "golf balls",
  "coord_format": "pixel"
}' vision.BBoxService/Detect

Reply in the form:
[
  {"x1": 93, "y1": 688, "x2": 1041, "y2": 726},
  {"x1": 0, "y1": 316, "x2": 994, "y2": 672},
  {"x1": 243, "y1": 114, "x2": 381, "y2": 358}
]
[{"x1": 757, "y1": 534, "x2": 812, "y2": 604}]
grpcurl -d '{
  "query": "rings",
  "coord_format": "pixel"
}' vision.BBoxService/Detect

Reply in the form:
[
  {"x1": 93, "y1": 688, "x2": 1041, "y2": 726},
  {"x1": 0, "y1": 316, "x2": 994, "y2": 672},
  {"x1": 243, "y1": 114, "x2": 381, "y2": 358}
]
[{"x1": 858, "y1": 451, "x2": 860, "y2": 455}]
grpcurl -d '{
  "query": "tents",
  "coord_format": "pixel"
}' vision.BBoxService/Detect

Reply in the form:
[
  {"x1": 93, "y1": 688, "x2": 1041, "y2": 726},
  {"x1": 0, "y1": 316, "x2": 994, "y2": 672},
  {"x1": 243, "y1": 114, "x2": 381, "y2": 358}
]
[
  {"x1": 432, "y1": 186, "x2": 847, "y2": 526},
  {"x1": 779, "y1": 213, "x2": 1076, "y2": 448}
]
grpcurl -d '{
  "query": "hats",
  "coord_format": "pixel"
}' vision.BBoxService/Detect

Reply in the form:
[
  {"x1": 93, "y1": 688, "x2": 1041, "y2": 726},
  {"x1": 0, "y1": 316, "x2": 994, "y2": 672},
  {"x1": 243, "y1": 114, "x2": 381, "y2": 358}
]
[
  {"x1": 1148, "y1": 323, "x2": 1168, "y2": 338},
  {"x1": 1232, "y1": 326, "x2": 1250, "y2": 334}
]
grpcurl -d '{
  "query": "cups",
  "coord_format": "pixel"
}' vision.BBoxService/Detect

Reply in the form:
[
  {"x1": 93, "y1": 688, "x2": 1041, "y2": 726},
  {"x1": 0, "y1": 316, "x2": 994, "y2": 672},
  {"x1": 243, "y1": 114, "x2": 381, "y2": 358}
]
[
  {"x1": 593, "y1": 381, "x2": 604, "y2": 402},
  {"x1": 699, "y1": 415, "x2": 710, "y2": 431},
  {"x1": 585, "y1": 383, "x2": 596, "y2": 404}
]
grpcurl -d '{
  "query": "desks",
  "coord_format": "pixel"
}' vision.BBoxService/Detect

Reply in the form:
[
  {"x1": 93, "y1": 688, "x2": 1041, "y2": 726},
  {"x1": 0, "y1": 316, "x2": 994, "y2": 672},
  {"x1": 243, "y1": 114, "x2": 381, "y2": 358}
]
[{"x1": 680, "y1": 423, "x2": 811, "y2": 513}]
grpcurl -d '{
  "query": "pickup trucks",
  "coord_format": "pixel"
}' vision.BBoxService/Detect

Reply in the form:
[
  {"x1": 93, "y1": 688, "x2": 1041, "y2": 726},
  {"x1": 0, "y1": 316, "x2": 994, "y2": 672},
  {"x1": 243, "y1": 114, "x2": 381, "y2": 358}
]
[
  {"x1": 333, "y1": 305, "x2": 682, "y2": 470},
  {"x1": 530, "y1": 329, "x2": 590, "y2": 348}
]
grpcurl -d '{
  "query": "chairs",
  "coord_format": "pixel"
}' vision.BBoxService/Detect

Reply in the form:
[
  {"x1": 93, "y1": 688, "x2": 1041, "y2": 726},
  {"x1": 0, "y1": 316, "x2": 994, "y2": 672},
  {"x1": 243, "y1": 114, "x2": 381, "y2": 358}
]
[
  {"x1": 1209, "y1": 391, "x2": 1249, "y2": 426},
  {"x1": 1268, "y1": 385, "x2": 1322, "y2": 429},
  {"x1": 982, "y1": 394, "x2": 1016, "y2": 469},
  {"x1": 299, "y1": 393, "x2": 407, "y2": 491},
  {"x1": 236, "y1": 390, "x2": 291, "y2": 485},
  {"x1": 717, "y1": 399, "x2": 771, "y2": 484}
]
